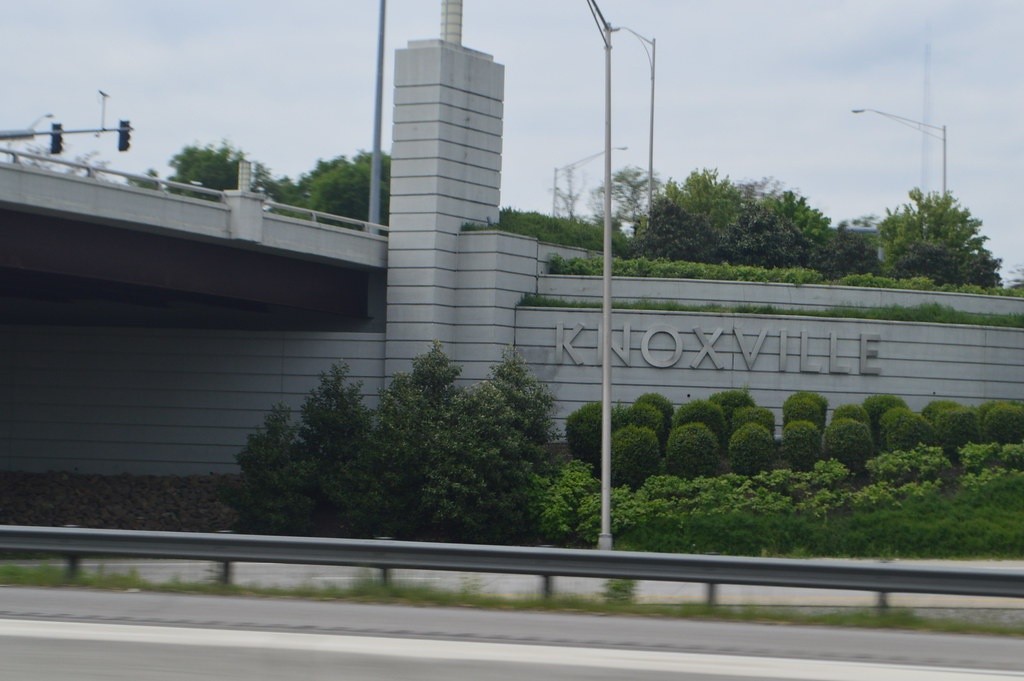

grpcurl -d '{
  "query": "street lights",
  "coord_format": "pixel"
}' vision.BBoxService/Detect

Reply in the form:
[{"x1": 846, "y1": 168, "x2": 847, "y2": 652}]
[
  {"x1": 605, "y1": 23, "x2": 657, "y2": 211},
  {"x1": 850, "y1": 105, "x2": 949, "y2": 197},
  {"x1": 551, "y1": 145, "x2": 628, "y2": 216}
]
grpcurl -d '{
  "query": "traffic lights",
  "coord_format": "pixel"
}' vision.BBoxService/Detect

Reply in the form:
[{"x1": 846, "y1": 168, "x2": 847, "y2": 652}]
[
  {"x1": 51, "y1": 123, "x2": 63, "y2": 154},
  {"x1": 118, "y1": 120, "x2": 131, "y2": 151}
]
